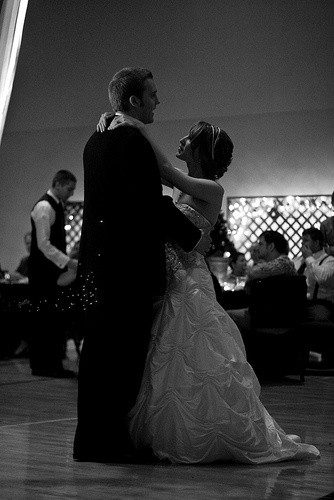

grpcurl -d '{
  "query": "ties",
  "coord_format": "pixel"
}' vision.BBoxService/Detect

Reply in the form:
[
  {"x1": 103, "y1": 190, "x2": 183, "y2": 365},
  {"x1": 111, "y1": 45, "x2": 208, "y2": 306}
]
[{"x1": 298, "y1": 263, "x2": 306, "y2": 274}]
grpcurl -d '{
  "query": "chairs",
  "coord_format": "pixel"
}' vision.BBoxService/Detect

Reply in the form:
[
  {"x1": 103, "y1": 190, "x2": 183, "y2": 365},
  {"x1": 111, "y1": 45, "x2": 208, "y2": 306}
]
[
  {"x1": 245, "y1": 275, "x2": 308, "y2": 383},
  {"x1": 305, "y1": 299, "x2": 334, "y2": 376}
]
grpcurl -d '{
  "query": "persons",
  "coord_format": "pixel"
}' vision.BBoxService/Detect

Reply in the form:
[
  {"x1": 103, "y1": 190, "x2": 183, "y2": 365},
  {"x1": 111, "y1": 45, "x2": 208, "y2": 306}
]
[
  {"x1": 97, "y1": 110, "x2": 321, "y2": 465},
  {"x1": 248, "y1": 231, "x2": 298, "y2": 278},
  {"x1": 30, "y1": 169, "x2": 76, "y2": 378},
  {"x1": 228, "y1": 252, "x2": 247, "y2": 282},
  {"x1": 293, "y1": 192, "x2": 334, "y2": 322},
  {"x1": 72, "y1": 66, "x2": 214, "y2": 463},
  {"x1": 14, "y1": 232, "x2": 32, "y2": 275},
  {"x1": 248, "y1": 242, "x2": 260, "y2": 268}
]
